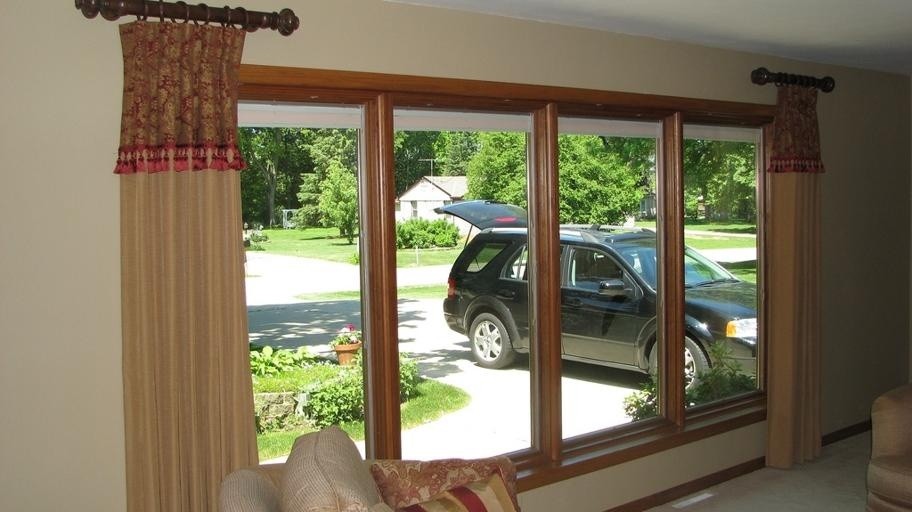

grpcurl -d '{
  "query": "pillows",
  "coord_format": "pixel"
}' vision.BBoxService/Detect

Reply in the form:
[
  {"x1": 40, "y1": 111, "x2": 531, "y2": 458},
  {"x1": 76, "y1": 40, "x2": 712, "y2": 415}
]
[{"x1": 279, "y1": 428, "x2": 520, "y2": 512}]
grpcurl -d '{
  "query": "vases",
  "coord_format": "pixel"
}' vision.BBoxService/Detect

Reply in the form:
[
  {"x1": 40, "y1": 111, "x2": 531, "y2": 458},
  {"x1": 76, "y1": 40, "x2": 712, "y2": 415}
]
[{"x1": 333, "y1": 340, "x2": 361, "y2": 366}]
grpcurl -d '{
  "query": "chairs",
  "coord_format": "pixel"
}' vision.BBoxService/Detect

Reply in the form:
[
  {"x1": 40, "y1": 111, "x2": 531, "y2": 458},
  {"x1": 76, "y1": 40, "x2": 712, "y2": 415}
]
[
  {"x1": 596, "y1": 254, "x2": 635, "y2": 280},
  {"x1": 216, "y1": 454, "x2": 522, "y2": 512},
  {"x1": 866, "y1": 384, "x2": 912, "y2": 511}
]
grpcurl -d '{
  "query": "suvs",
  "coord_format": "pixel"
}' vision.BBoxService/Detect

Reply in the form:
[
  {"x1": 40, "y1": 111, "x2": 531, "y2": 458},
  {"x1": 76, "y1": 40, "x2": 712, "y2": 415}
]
[{"x1": 431, "y1": 195, "x2": 759, "y2": 400}]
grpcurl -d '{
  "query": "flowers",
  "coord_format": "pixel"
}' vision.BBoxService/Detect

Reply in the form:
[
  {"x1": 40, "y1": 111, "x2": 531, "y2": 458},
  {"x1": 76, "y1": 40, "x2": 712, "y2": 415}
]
[{"x1": 327, "y1": 323, "x2": 363, "y2": 350}]
